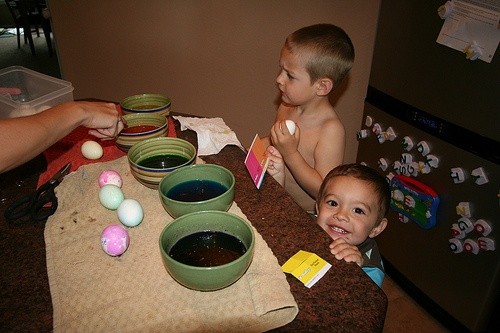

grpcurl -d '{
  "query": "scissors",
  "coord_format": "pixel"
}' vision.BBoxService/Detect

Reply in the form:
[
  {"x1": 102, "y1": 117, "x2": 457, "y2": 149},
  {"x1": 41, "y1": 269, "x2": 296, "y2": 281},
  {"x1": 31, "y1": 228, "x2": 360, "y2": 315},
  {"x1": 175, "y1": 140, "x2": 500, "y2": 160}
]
[{"x1": 3, "y1": 161, "x2": 72, "y2": 222}]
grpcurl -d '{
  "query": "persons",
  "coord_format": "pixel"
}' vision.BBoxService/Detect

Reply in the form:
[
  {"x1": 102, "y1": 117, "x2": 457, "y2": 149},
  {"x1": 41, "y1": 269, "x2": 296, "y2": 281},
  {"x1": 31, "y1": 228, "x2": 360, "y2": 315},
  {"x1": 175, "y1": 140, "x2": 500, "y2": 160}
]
[
  {"x1": 0, "y1": 101, "x2": 127, "y2": 174},
  {"x1": 245, "y1": 22, "x2": 355, "y2": 216},
  {"x1": 262, "y1": 146, "x2": 392, "y2": 291}
]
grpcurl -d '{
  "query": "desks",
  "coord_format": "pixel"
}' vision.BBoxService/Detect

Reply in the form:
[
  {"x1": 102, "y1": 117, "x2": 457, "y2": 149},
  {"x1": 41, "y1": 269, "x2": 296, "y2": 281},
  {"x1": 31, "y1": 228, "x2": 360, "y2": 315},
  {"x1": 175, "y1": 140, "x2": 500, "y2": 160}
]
[{"x1": 0, "y1": 98, "x2": 389, "y2": 333}]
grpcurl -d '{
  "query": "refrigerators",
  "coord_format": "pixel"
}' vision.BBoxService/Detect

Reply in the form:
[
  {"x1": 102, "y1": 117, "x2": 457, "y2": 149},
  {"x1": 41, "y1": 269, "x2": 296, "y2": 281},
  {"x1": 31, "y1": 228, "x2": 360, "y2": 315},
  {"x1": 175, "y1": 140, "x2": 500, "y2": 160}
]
[{"x1": 354, "y1": 0, "x2": 500, "y2": 332}]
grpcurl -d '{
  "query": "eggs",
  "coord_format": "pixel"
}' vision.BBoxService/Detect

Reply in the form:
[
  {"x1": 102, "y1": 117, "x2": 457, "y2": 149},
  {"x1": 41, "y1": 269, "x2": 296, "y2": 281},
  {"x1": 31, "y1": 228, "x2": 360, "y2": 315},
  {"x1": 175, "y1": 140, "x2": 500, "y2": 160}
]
[
  {"x1": 81, "y1": 141, "x2": 103, "y2": 159},
  {"x1": 117, "y1": 198, "x2": 144, "y2": 227},
  {"x1": 99, "y1": 184, "x2": 124, "y2": 210},
  {"x1": 279, "y1": 119, "x2": 296, "y2": 135},
  {"x1": 98, "y1": 170, "x2": 123, "y2": 188},
  {"x1": 101, "y1": 224, "x2": 129, "y2": 256}
]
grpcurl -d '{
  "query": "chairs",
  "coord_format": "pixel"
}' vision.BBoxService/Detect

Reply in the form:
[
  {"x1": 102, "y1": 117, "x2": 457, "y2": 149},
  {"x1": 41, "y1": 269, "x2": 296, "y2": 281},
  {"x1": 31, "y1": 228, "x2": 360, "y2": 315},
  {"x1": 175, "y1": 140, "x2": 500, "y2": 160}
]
[{"x1": 4, "y1": 0, "x2": 53, "y2": 58}]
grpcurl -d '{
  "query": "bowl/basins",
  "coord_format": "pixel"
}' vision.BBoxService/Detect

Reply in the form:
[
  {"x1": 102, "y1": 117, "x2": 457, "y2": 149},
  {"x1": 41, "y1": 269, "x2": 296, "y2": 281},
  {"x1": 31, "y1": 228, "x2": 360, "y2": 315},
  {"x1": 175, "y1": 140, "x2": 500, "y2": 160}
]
[
  {"x1": 114, "y1": 112, "x2": 168, "y2": 153},
  {"x1": 158, "y1": 164, "x2": 235, "y2": 219},
  {"x1": 127, "y1": 136, "x2": 197, "y2": 190},
  {"x1": 119, "y1": 93, "x2": 171, "y2": 117},
  {"x1": 159, "y1": 210, "x2": 256, "y2": 292}
]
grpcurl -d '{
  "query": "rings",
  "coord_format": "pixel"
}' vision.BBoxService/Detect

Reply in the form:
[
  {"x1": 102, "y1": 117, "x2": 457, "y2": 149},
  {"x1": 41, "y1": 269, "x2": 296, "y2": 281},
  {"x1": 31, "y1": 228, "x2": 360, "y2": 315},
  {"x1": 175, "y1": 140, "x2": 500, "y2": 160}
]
[{"x1": 118, "y1": 118, "x2": 122, "y2": 123}]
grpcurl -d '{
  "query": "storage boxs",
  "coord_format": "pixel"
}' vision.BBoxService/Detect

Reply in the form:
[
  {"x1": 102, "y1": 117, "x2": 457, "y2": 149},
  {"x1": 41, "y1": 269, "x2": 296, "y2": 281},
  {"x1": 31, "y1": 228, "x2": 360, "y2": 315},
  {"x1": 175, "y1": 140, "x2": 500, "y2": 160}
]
[{"x1": 0, "y1": 66, "x2": 75, "y2": 119}]
